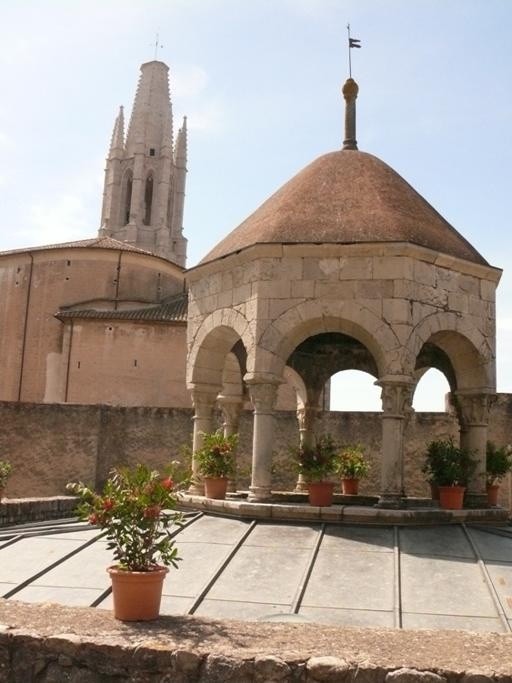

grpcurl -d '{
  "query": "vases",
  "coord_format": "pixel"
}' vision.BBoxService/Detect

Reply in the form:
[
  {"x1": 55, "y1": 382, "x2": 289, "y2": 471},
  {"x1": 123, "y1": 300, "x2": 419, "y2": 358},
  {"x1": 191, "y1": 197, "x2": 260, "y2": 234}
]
[
  {"x1": 308, "y1": 482, "x2": 332, "y2": 506},
  {"x1": 106, "y1": 566, "x2": 169, "y2": 621},
  {"x1": 341, "y1": 479, "x2": 359, "y2": 495},
  {"x1": 205, "y1": 478, "x2": 227, "y2": 499}
]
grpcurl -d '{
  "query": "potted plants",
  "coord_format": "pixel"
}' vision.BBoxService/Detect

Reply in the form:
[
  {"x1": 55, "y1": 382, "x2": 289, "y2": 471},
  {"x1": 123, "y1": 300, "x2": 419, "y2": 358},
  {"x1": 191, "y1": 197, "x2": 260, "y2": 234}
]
[
  {"x1": 486, "y1": 439, "x2": 512, "y2": 506},
  {"x1": 424, "y1": 433, "x2": 481, "y2": 510},
  {"x1": 334, "y1": 444, "x2": 372, "y2": 496}
]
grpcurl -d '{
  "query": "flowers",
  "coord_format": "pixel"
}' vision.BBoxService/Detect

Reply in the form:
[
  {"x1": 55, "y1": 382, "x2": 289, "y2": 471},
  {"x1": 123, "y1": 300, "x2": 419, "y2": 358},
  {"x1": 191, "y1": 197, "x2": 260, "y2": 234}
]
[
  {"x1": 191, "y1": 427, "x2": 241, "y2": 478},
  {"x1": 287, "y1": 434, "x2": 348, "y2": 483},
  {"x1": 66, "y1": 460, "x2": 202, "y2": 570}
]
